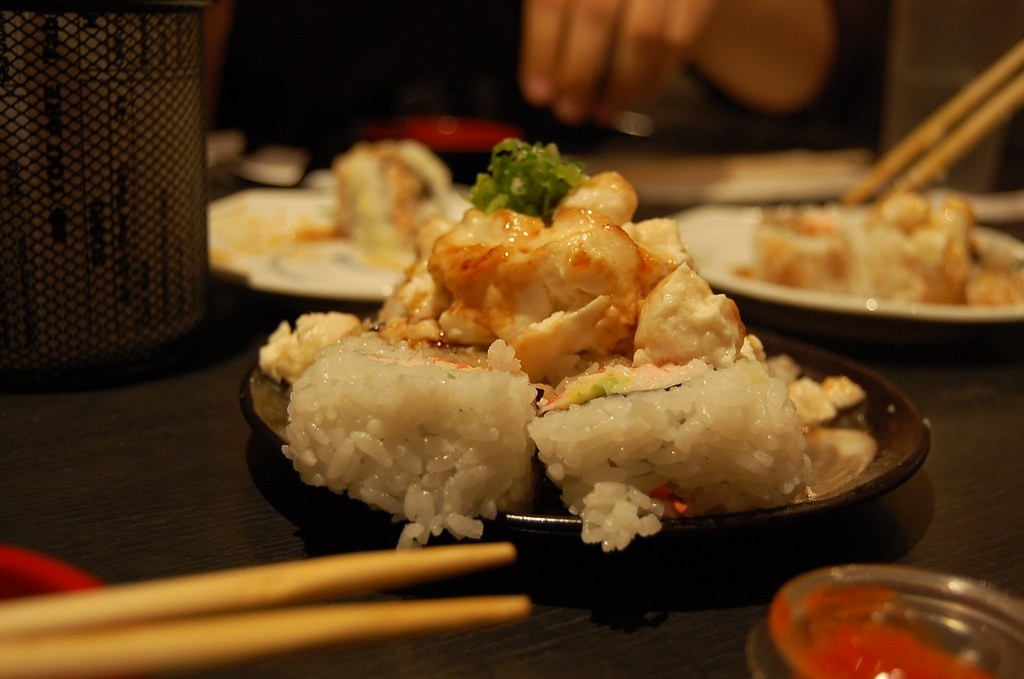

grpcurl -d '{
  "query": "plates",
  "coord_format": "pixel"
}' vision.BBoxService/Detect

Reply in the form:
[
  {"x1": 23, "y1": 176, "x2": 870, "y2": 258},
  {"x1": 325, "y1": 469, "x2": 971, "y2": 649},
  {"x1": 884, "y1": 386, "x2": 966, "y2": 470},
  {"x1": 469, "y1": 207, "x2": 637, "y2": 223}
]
[
  {"x1": 240, "y1": 320, "x2": 931, "y2": 537},
  {"x1": 671, "y1": 206, "x2": 1024, "y2": 324},
  {"x1": 747, "y1": 565, "x2": 1024, "y2": 679},
  {"x1": 203, "y1": 191, "x2": 475, "y2": 301}
]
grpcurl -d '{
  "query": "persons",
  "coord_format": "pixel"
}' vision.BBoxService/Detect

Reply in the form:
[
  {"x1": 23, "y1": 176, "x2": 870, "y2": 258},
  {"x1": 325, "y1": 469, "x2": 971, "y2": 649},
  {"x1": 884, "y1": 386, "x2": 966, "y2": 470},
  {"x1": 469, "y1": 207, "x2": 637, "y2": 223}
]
[{"x1": 206, "y1": 0, "x2": 895, "y2": 186}]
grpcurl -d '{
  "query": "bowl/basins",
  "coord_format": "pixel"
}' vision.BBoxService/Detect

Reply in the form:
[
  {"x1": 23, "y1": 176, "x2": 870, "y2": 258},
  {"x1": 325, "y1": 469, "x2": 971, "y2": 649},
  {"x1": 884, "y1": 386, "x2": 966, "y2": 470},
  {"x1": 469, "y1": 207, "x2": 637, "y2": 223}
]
[{"x1": 0, "y1": 545, "x2": 106, "y2": 603}]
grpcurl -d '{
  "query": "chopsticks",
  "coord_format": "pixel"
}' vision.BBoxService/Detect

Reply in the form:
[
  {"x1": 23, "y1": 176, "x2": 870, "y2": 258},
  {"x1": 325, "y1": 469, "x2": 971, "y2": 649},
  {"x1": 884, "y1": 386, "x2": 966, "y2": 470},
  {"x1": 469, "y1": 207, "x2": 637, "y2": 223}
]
[
  {"x1": 0, "y1": 541, "x2": 530, "y2": 679},
  {"x1": 841, "y1": 39, "x2": 1024, "y2": 210}
]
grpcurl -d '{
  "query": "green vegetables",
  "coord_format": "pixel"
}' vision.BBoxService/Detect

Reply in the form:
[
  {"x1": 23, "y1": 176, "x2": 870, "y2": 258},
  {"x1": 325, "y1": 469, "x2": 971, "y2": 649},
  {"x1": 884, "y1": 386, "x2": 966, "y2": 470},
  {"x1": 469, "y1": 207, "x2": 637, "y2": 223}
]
[{"x1": 463, "y1": 136, "x2": 586, "y2": 229}]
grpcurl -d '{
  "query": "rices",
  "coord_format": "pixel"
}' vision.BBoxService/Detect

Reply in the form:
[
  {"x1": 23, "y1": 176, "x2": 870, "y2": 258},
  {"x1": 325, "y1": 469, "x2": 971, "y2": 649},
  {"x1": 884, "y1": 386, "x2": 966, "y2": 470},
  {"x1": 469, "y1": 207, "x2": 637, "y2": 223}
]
[{"x1": 279, "y1": 332, "x2": 814, "y2": 554}]
